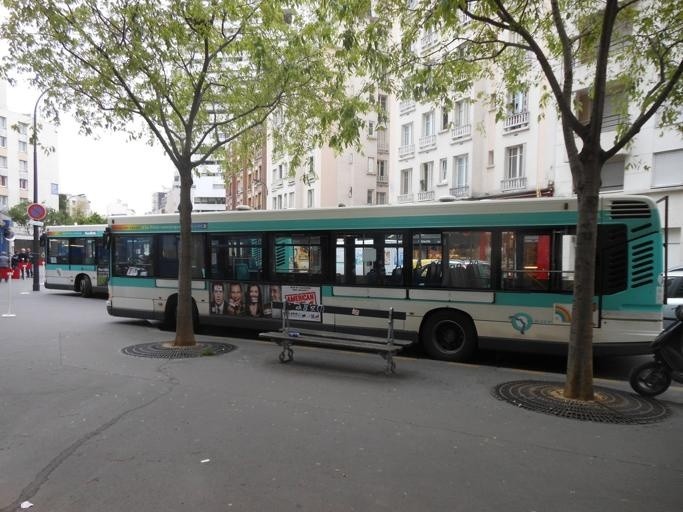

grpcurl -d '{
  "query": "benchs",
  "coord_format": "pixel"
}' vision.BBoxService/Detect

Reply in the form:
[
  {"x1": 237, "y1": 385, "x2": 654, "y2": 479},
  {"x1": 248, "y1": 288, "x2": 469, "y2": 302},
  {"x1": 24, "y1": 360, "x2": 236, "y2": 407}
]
[{"x1": 258, "y1": 299, "x2": 413, "y2": 378}]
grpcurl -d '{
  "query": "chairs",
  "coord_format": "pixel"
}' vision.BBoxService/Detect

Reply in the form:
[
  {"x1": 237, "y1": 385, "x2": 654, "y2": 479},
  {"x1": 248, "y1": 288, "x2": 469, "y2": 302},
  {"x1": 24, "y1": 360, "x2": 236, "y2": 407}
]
[{"x1": 366, "y1": 260, "x2": 490, "y2": 290}]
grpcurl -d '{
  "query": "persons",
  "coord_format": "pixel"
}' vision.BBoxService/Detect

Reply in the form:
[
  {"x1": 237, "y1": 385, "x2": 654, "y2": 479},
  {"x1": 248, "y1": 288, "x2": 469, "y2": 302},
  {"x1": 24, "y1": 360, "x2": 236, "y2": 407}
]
[
  {"x1": 207, "y1": 283, "x2": 227, "y2": 315},
  {"x1": 224, "y1": 282, "x2": 242, "y2": 316},
  {"x1": 243, "y1": 284, "x2": 261, "y2": 318},
  {"x1": 0, "y1": 247, "x2": 33, "y2": 283},
  {"x1": 261, "y1": 285, "x2": 280, "y2": 316}
]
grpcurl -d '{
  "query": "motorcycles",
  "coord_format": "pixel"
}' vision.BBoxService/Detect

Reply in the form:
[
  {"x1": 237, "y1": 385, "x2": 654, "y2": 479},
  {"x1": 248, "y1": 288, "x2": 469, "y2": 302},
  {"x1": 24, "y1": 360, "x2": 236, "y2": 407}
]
[{"x1": 629, "y1": 305, "x2": 682, "y2": 395}]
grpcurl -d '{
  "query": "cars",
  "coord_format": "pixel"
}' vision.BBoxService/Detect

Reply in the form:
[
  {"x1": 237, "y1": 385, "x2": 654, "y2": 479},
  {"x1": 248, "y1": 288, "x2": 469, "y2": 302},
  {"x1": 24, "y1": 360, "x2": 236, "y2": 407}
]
[{"x1": 663, "y1": 268, "x2": 683, "y2": 329}]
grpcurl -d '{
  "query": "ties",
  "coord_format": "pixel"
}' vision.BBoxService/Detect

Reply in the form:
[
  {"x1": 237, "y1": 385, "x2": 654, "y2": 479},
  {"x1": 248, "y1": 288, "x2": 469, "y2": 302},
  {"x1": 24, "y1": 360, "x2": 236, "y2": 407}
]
[{"x1": 216, "y1": 305, "x2": 221, "y2": 315}]
